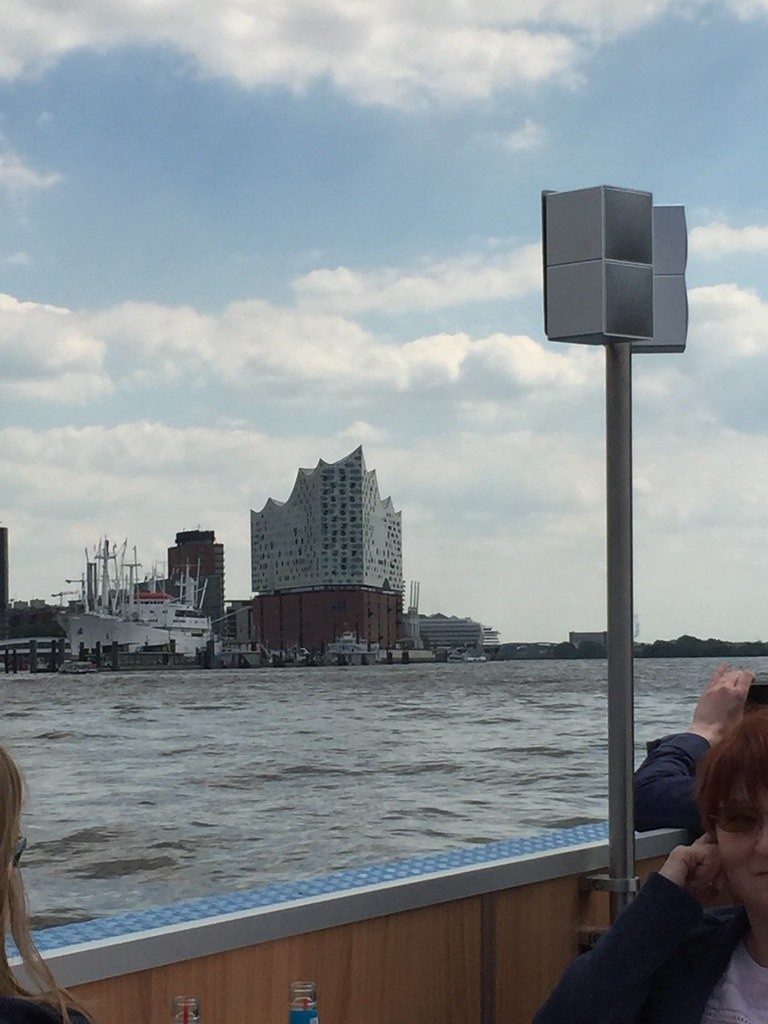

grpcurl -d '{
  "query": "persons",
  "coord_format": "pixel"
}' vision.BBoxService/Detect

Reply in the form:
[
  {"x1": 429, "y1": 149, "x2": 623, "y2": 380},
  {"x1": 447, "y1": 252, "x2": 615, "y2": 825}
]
[
  {"x1": 0, "y1": 747, "x2": 96, "y2": 1024},
  {"x1": 532, "y1": 662, "x2": 768, "y2": 1024}
]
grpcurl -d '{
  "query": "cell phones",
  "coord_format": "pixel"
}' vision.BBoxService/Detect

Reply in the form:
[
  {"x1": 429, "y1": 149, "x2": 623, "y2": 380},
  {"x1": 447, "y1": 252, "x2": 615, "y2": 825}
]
[{"x1": 748, "y1": 684, "x2": 768, "y2": 705}]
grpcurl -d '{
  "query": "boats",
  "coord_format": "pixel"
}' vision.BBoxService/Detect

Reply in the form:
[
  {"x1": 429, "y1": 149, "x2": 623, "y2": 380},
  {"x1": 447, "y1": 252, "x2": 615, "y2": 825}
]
[{"x1": 50, "y1": 533, "x2": 213, "y2": 657}]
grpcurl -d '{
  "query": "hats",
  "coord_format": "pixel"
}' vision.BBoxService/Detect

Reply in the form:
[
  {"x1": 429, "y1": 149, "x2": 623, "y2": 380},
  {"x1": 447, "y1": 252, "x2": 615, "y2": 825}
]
[{"x1": 751, "y1": 671, "x2": 768, "y2": 685}]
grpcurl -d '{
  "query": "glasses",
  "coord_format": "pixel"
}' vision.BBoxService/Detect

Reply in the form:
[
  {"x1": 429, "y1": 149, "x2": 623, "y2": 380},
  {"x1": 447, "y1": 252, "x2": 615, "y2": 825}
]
[
  {"x1": 12, "y1": 834, "x2": 27, "y2": 866},
  {"x1": 709, "y1": 803, "x2": 768, "y2": 834}
]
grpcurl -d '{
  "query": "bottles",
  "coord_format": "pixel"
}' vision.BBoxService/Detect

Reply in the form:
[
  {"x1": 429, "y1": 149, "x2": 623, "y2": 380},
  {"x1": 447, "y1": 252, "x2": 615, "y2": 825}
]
[
  {"x1": 288, "y1": 980, "x2": 319, "y2": 1024},
  {"x1": 169, "y1": 995, "x2": 200, "y2": 1024}
]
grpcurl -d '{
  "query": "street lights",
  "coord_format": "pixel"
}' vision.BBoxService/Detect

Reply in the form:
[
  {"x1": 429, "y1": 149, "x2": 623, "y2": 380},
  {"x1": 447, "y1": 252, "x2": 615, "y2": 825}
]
[{"x1": 537, "y1": 181, "x2": 687, "y2": 938}]
